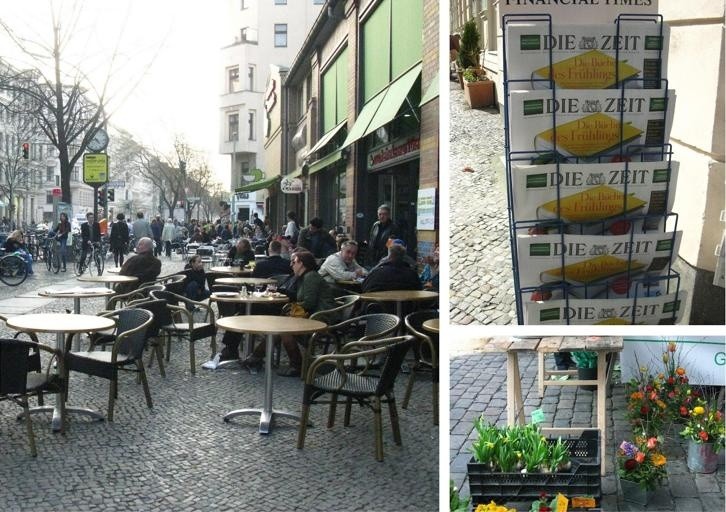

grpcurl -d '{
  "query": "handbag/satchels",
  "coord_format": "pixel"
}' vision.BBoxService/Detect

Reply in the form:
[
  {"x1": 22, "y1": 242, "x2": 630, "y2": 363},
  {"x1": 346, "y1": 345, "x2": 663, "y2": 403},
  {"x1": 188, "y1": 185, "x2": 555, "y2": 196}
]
[{"x1": 65, "y1": 231, "x2": 74, "y2": 247}]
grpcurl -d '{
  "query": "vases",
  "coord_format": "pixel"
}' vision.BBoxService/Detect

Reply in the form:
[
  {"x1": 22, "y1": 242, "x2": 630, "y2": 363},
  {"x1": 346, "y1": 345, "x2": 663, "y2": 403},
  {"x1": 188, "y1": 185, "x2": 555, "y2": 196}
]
[
  {"x1": 617, "y1": 474, "x2": 659, "y2": 508},
  {"x1": 685, "y1": 436, "x2": 720, "y2": 475}
]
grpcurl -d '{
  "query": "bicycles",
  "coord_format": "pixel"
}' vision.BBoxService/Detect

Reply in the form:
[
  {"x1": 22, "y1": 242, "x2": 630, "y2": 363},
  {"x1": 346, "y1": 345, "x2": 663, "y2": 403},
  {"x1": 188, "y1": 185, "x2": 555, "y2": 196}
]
[{"x1": 0, "y1": 219, "x2": 184, "y2": 288}]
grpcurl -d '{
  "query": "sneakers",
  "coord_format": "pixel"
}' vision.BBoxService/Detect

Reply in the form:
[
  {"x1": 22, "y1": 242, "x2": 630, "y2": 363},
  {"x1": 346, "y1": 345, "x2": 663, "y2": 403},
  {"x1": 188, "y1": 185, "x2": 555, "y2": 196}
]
[
  {"x1": 277, "y1": 368, "x2": 305, "y2": 378},
  {"x1": 238, "y1": 356, "x2": 263, "y2": 367},
  {"x1": 215, "y1": 348, "x2": 239, "y2": 361}
]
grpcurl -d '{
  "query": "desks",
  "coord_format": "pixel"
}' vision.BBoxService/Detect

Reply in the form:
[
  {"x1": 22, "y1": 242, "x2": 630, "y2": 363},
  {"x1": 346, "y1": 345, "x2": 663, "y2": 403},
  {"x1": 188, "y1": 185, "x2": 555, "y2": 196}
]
[{"x1": 502, "y1": 337, "x2": 625, "y2": 478}]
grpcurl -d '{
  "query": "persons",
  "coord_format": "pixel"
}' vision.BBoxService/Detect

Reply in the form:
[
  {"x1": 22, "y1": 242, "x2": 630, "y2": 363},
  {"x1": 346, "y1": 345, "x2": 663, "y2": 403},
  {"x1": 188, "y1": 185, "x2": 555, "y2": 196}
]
[
  {"x1": 4, "y1": 229, "x2": 35, "y2": 275},
  {"x1": 0, "y1": 215, "x2": 48, "y2": 231},
  {"x1": 367, "y1": 203, "x2": 398, "y2": 257},
  {"x1": 41, "y1": 212, "x2": 70, "y2": 272},
  {"x1": 77, "y1": 212, "x2": 102, "y2": 275}
]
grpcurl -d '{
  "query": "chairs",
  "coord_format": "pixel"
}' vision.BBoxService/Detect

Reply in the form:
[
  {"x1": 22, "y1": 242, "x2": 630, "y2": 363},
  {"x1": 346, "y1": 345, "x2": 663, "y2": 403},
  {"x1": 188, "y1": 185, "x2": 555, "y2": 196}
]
[{"x1": 0, "y1": 238, "x2": 439, "y2": 466}]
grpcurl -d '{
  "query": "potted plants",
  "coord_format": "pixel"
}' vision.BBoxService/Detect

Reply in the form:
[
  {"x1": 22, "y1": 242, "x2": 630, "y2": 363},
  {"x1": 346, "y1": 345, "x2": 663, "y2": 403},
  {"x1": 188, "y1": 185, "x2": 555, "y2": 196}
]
[
  {"x1": 672, "y1": 417, "x2": 689, "y2": 437},
  {"x1": 455, "y1": 26, "x2": 495, "y2": 110},
  {"x1": 573, "y1": 348, "x2": 600, "y2": 391},
  {"x1": 552, "y1": 351, "x2": 572, "y2": 371}
]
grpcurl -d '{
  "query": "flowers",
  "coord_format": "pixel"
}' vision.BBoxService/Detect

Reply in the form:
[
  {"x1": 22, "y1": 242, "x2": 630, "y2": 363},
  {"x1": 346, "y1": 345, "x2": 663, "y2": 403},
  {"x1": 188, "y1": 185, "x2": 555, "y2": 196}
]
[
  {"x1": 469, "y1": 417, "x2": 572, "y2": 478},
  {"x1": 681, "y1": 398, "x2": 725, "y2": 443},
  {"x1": 658, "y1": 342, "x2": 698, "y2": 417},
  {"x1": 623, "y1": 359, "x2": 665, "y2": 425},
  {"x1": 617, "y1": 425, "x2": 666, "y2": 478},
  {"x1": 472, "y1": 498, "x2": 517, "y2": 512},
  {"x1": 527, "y1": 490, "x2": 568, "y2": 512}
]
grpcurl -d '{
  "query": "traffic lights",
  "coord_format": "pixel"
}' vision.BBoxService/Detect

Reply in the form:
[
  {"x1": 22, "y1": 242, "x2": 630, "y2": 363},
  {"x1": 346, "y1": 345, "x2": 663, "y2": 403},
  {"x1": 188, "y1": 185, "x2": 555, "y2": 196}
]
[
  {"x1": 106, "y1": 189, "x2": 113, "y2": 202},
  {"x1": 97, "y1": 188, "x2": 103, "y2": 207},
  {"x1": 21, "y1": 142, "x2": 28, "y2": 160}
]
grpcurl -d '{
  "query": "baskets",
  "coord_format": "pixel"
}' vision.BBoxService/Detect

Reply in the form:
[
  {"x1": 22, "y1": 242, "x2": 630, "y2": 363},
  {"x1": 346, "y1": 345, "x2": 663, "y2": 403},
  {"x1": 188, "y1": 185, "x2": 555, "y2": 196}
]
[{"x1": 466, "y1": 429, "x2": 603, "y2": 510}]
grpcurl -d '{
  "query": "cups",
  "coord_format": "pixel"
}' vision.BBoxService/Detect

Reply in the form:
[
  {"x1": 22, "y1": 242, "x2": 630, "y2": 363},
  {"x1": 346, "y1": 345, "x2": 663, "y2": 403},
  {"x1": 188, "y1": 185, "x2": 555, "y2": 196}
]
[{"x1": 226, "y1": 258, "x2": 281, "y2": 297}]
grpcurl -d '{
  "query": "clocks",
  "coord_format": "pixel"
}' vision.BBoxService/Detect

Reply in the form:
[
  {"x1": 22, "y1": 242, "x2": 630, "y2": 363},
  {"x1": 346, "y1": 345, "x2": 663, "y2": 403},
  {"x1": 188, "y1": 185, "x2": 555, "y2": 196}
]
[{"x1": 85, "y1": 127, "x2": 110, "y2": 152}]
